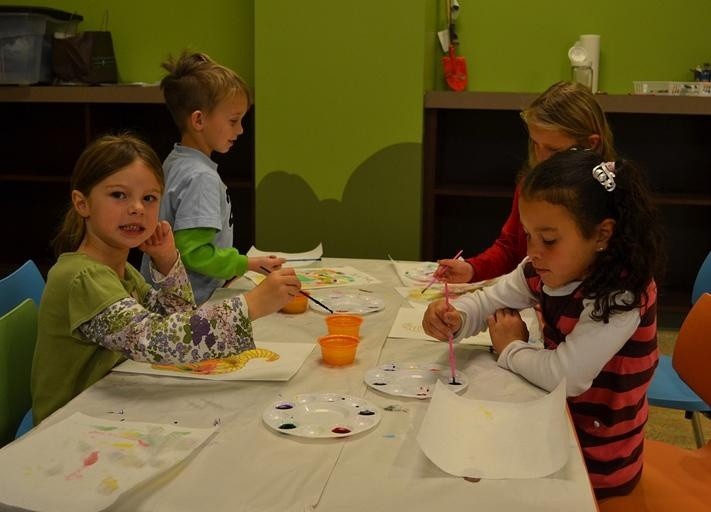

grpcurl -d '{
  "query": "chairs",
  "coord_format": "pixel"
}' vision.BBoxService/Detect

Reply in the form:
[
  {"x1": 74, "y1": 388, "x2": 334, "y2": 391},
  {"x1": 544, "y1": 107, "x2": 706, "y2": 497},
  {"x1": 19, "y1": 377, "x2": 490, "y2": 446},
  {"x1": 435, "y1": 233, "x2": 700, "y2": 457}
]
[
  {"x1": 574, "y1": 251, "x2": 711, "y2": 512},
  {"x1": 0, "y1": 260, "x2": 45, "y2": 445}
]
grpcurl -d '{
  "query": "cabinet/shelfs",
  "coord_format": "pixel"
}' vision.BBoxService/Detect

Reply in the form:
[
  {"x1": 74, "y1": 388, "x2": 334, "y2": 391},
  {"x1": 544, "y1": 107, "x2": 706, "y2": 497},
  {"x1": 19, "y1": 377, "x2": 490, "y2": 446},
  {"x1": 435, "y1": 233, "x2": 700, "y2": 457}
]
[
  {"x1": 421, "y1": 86, "x2": 709, "y2": 328},
  {"x1": 3, "y1": 84, "x2": 253, "y2": 286}
]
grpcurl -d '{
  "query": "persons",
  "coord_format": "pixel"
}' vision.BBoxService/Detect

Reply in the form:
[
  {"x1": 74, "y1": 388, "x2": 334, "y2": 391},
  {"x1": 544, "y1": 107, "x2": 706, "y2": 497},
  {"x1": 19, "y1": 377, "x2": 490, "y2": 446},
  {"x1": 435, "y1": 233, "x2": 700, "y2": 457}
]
[
  {"x1": 137, "y1": 45, "x2": 287, "y2": 314},
  {"x1": 421, "y1": 147, "x2": 660, "y2": 501},
  {"x1": 27, "y1": 134, "x2": 303, "y2": 430},
  {"x1": 431, "y1": 77, "x2": 614, "y2": 284}
]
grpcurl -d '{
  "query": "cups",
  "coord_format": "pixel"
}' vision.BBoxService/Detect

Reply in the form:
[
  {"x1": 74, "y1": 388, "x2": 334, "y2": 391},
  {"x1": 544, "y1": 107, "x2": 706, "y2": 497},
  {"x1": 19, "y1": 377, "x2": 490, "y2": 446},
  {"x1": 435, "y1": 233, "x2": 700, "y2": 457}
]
[
  {"x1": 282, "y1": 288, "x2": 309, "y2": 314},
  {"x1": 318, "y1": 312, "x2": 362, "y2": 369}
]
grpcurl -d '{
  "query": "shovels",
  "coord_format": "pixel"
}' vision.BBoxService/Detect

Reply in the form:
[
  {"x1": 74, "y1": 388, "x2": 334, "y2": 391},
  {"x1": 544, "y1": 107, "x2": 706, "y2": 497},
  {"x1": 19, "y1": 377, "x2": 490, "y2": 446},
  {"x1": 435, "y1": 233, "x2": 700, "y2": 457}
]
[{"x1": 441, "y1": 0, "x2": 468, "y2": 91}]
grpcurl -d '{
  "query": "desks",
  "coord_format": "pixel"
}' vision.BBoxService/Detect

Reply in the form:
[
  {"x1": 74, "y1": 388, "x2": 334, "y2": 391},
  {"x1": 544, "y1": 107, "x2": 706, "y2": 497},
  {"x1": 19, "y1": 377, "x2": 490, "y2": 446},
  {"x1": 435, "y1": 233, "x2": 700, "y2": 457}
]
[{"x1": 0, "y1": 260, "x2": 597, "y2": 510}]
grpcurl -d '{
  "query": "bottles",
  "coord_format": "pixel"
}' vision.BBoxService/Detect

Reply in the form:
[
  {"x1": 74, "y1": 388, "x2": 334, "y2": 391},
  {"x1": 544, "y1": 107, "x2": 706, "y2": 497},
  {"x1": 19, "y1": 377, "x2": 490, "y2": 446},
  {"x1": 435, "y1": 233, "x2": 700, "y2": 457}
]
[{"x1": 569, "y1": 62, "x2": 593, "y2": 92}]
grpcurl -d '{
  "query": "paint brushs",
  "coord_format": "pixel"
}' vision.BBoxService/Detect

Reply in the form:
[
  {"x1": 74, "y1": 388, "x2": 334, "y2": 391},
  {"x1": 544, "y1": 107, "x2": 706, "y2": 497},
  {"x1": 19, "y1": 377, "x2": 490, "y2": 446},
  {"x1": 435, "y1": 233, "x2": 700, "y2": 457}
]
[
  {"x1": 421, "y1": 249, "x2": 464, "y2": 294},
  {"x1": 445, "y1": 281, "x2": 457, "y2": 384},
  {"x1": 259, "y1": 266, "x2": 334, "y2": 314},
  {"x1": 285, "y1": 259, "x2": 321, "y2": 261}
]
